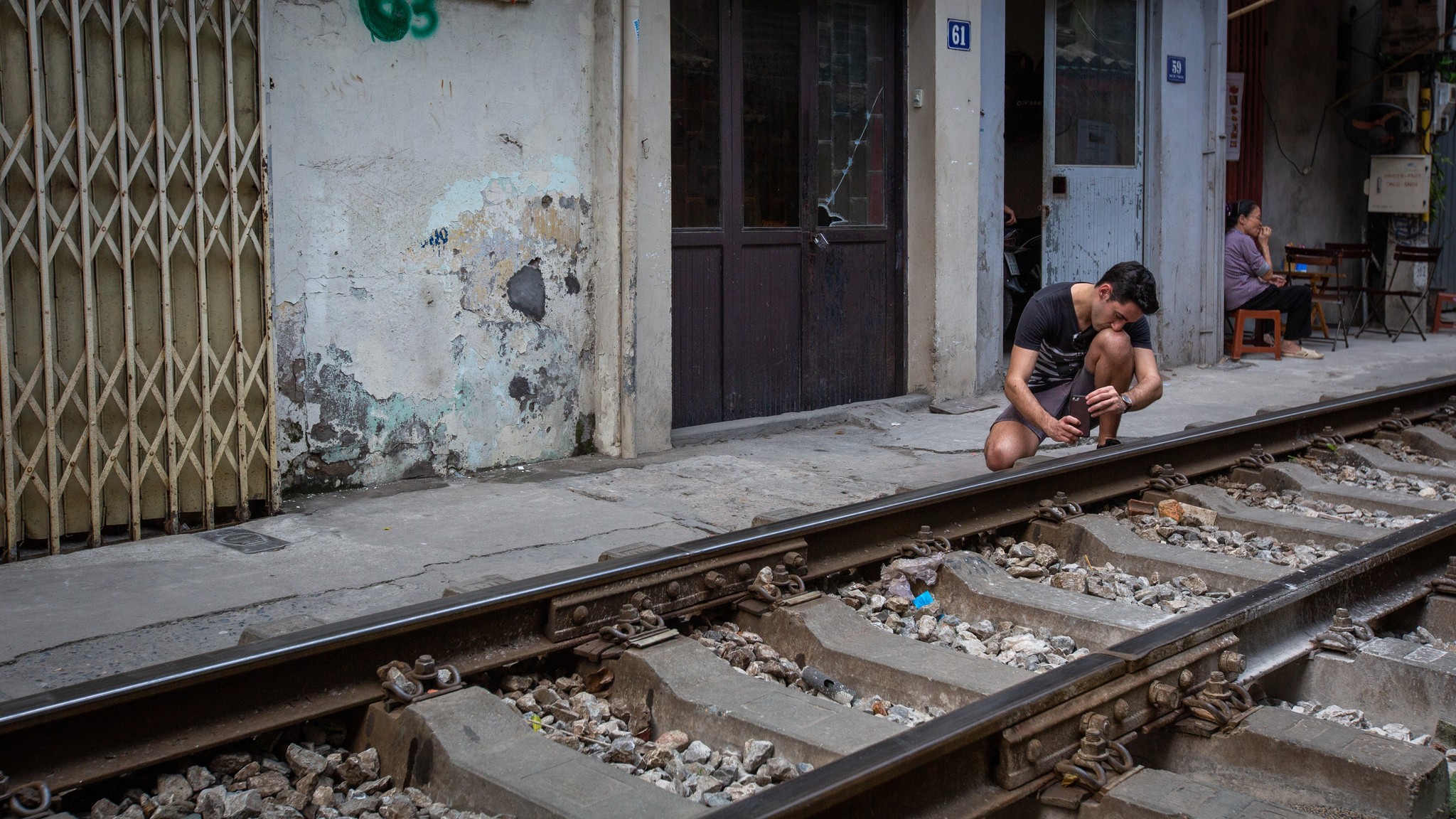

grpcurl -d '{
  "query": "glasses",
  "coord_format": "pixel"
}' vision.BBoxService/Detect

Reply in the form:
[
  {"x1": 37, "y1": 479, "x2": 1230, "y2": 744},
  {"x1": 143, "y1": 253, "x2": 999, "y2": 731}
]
[{"x1": 1070, "y1": 330, "x2": 1087, "y2": 369}]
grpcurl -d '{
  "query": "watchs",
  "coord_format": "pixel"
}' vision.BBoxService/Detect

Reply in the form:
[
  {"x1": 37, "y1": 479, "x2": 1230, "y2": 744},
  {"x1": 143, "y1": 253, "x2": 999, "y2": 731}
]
[{"x1": 1118, "y1": 394, "x2": 1132, "y2": 414}]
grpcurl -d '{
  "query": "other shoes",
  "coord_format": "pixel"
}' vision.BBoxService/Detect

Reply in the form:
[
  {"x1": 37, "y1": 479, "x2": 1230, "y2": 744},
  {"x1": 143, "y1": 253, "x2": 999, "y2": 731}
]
[{"x1": 1097, "y1": 437, "x2": 1120, "y2": 448}]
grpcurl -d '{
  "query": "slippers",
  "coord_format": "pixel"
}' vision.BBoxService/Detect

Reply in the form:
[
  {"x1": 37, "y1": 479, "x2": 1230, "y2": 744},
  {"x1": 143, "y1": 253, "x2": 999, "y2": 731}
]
[{"x1": 1281, "y1": 345, "x2": 1323, "y2": 360}]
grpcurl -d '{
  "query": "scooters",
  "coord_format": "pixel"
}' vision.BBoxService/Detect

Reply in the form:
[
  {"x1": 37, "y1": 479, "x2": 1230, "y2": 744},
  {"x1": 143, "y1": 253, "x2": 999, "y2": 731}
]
[{"x1": 1002, "y1": 225, "x2": 1042, "y2": 339}]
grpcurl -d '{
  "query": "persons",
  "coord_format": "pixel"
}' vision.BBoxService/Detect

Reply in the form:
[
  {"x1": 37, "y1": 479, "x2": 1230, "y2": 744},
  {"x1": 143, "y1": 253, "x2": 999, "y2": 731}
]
[
  {"x1": 984, "y1": 262, "x2": 1162, "y2": 473},
  {"x1": 1003, "y1": 198, "x2": 1016, "y2": 225},
  {"x1": 1224, "y1": 200, "x2": 1324, "y2": 359}
]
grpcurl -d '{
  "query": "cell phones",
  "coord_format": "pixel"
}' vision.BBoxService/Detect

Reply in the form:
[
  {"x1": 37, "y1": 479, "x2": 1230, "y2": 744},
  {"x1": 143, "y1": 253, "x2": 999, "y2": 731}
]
[{"x1": 1070, "y1": 395, "x2": 1092, "y2": 438}]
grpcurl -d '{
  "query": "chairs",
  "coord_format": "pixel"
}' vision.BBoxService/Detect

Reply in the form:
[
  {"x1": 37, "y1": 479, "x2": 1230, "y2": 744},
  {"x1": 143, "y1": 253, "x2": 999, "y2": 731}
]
[{"x1": 1283, "y1": 243, "x2": 1442, "y2": 352}]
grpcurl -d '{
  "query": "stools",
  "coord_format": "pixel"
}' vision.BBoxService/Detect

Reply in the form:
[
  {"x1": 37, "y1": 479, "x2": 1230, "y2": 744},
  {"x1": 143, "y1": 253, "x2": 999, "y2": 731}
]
[
  {"x1": 1433, "y1": 291, "x2": 1456, "y2": 333},
  {"x1": 1223, "y1": 309, "x2": 1282, "y2": 361}
]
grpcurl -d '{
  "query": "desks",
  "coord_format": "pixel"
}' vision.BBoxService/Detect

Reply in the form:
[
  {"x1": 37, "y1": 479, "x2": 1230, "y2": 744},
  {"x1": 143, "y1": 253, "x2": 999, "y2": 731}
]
[{"x1": 1266, "y1": 271, "x2": 1347, "y2": 341}]
